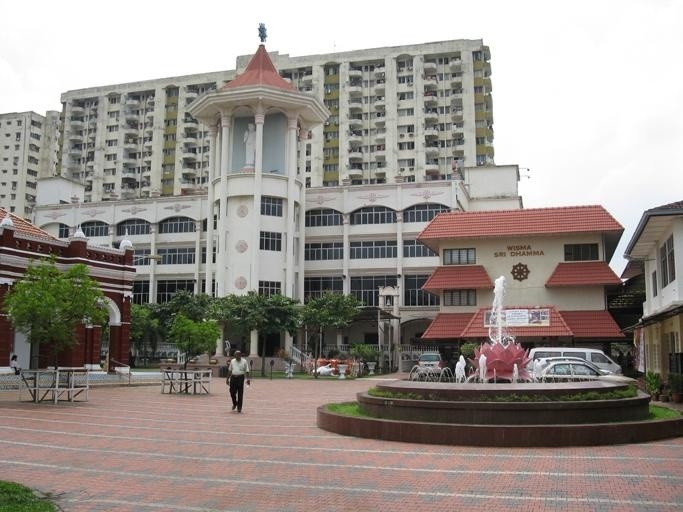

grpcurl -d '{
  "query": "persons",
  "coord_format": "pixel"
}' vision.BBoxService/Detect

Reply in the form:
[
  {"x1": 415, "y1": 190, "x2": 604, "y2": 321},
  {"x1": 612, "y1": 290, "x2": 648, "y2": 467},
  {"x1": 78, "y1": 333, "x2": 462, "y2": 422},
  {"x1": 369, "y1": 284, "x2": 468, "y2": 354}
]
[
  {"x1": 243, "y1": 122, "x2": 256, "y2": 165},
  {"x1": 226, "y1": 350, "x2": 250, "y2": 413},
  {"x1": 10, "y1": 355, "x2": 22, "y2": 375}
]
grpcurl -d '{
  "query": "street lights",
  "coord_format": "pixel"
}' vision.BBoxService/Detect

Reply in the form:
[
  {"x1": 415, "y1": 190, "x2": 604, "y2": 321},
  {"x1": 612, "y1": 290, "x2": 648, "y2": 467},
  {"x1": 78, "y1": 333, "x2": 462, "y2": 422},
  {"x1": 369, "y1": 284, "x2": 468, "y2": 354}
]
[
  {"x1": 520, "y1": 167, "x2": 531, "y2": 180},
  {"x1": 386, "y1": 298, "x2": 391, "y2": 373}
]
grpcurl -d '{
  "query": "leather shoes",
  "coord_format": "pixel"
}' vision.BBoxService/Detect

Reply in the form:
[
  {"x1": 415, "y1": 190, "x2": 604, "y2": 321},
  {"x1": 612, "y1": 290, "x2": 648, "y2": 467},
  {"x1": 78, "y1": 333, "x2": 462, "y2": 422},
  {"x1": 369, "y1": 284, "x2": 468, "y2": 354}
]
[{"x1": 232, "y1": 401, "x2": 241, "y2": 412}]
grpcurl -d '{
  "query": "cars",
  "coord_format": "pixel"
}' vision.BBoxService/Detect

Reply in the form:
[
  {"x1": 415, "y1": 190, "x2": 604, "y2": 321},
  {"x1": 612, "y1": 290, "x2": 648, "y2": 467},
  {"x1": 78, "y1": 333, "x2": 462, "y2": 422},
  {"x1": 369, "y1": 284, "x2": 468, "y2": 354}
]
[
  {"x1": 526, "y1": 347, "x2": 639, "y2": 385},
  {"x1": 417, "y1": 354, "x2": 442, "y2": 378}
]
